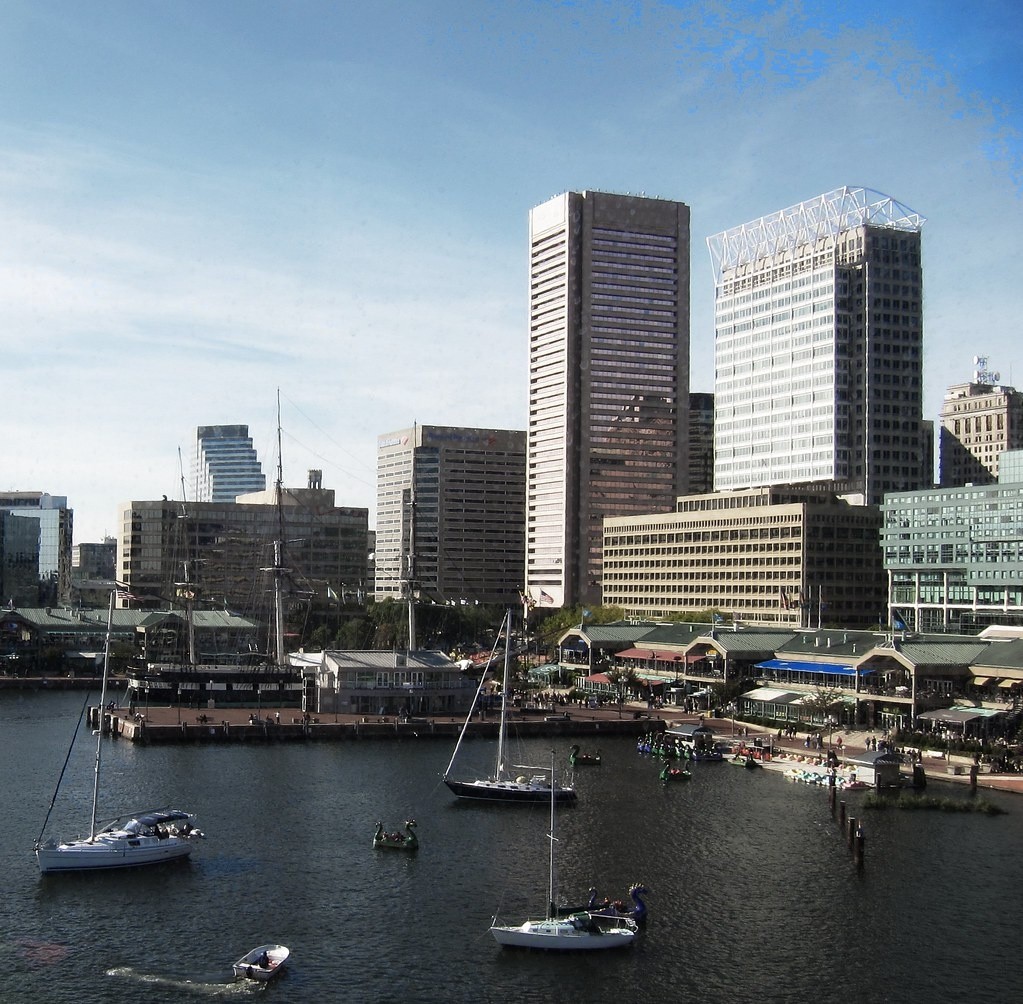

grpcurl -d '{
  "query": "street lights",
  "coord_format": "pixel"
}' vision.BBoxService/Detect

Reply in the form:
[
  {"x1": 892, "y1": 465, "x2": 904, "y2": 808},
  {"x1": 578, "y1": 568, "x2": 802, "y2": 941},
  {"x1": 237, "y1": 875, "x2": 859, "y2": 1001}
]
[
  {"x1": 480, "y1": 691, "x2": 484, "y2": 712},
  {"x1": 941, "y1": 730, "x2": 956, "y2": 766},
  {"x1": 824, "y1": 715, "x2": 837, "y2": 746},
  {"x1": 618, "y1": 675, "x2": 628, "y2": 698},
  {"x1": 409, "y1": 689, "x2": 413, "y2": 715},
  {"x1": 210, "y1": 679, "x2": 213, "y2": 700},
  {"x1": 726, "y1": 702, "x2": 738, "y2": 737},
  {"x1": 257, "y1": 690, "x2": 261, "y2": 719},
  {"x1": 335, "y1": 690, "x2": 339, "y2": 723},
  {"x1": 704, "y1": 685, "x2": 713, "y2": 708},
  {"x1": 178, "y1": 688, "x2": 181, "y2": 723},
  {"x1": 145, "y1": 688, "x2": 149, "y2": 720}
]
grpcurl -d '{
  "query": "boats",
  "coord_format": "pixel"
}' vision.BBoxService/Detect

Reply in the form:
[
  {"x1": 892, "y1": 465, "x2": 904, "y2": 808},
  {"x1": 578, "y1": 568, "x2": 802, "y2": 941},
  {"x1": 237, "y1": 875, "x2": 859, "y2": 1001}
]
[
  {"x1": 728, "y1": 749, "x2": 758, "y2": 769},
  {"x1": 660, "y1": 759, "x2": 692, "y2": 782},
  {"x1": 558, "y1": 883, "x2": 648, "y2": 926},
  {"x1": 783, "y1": 769, "x2": 871, "y2": 790},
  {"x1": 373, "y1": 820, "x2": 420, "y2": 850},
  {"x1": 569, "y1": 744, "x2": 603, "y2": 766},
  {"x1": 637, "y1": 730, "x2": 724, "y2": 762},
  {"x1": 232, "y1": 944, "x2": 290, "y2": 981}
]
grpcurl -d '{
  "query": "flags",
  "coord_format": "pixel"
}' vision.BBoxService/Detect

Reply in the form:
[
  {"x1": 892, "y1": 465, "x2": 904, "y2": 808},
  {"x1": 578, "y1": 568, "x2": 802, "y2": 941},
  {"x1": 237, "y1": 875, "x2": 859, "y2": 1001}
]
[
  {"x1": 781, "y1": 591, "x2": 826, "y2": 611},
  {"x1": 519, "y1": 586, "x2": 554, "y2": 612},
  {"x1": 733, "y1": 612, "x2": 739, "y2": 620},
  {"x1": 583, "y1": 609, "x2": 592, "y2": 617},
  {"x1": 715, "y1": 614, "x2": 724, "y2": 621}
]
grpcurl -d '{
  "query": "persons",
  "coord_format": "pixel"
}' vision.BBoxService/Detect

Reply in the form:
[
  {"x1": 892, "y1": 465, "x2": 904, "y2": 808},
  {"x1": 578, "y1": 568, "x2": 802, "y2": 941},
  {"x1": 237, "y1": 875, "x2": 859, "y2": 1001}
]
[
  {"x1": 381, "y1": 831, "x2": 403, "y2": 842},
  {"x1": 107, "y1": 701, "x2": 145, "y2": 722},
  {"x1": 200, "y1": 710, "x2": 310, "y2": 725},
  {"x1": 737, "y1": 724, "x2": 842, "y2": 750},
  {"x1": 154, "y1": 822, "x2": 194, "y2": 841},
  {"x1": 484, "y1": 683, "x2": 705, "y2": 726},
  {"x1": 258, "y1": 951, "x2": 269, "y2": 968},
  {"x1": 865, "y1": 736, "x2": 1023, "y2": 774}
]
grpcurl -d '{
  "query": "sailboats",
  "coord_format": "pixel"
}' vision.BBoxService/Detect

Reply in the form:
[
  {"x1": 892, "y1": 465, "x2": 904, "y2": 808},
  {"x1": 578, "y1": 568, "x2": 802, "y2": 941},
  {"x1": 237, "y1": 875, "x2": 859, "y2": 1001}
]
[
  {"x1": 33, "y1": 592, "x2": 207, "y2": 873},
  {"x1": 490, "y1": 749, "x2": 637, "y2": 949},
  {"x1": 442, "y1": 608, "x2": 578, "y2": 804},
  {"x1": 124, "y1": 385, "x2": 571, "y2": 709}
]
